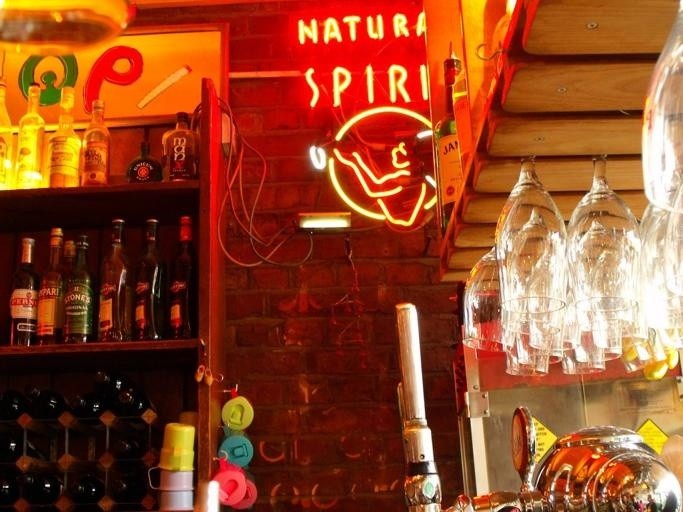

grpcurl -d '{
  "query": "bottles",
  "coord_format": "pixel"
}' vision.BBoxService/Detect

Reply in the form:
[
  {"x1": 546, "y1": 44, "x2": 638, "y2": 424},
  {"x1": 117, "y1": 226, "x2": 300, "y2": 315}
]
[
  {"x1": 162, "y1": 112, "x2": 194, "y2": 180},
  {"x1": 431, "y1": 60, "x2": 473, "y2": 238},
  {"x1": 97, "y1": 218, "x2": 130, "y2": 341},
  {"x1": 63, "y1": 240, "x2": 72, "y2": 277},
  {"x1": 17, "y1": 83, "x2": 45, "y2": 189},
  {"x1": 0, "y1": 369, "x2": 151, "y2": 511},
  {"x1": 167, "y1": 214, "x2": 199, "y2": 339},
  {"x1": 36, "y1": 227, "x2": 62, "y2": 345},
  {"x1": 0, "y1": 84, "x2": 14, "y2": 188},
  {"x1": 132, "y1": 218, "x2": 166, "y2": 341},
  {"x1": 10, "y1": 237, "x2": 38, "y2": 346},
  {"x1": 48, "y1": 86, "x2": 81, "y2": 189},
  {"x1": 128, "y1": 144, "x2": 161, "y2": 183},
  {"x1": 61, "y1": 235, "x2": 91, "y2": 343},
  {"x1": 82, "y1": 99, "x2": 108, "y2": 187}
]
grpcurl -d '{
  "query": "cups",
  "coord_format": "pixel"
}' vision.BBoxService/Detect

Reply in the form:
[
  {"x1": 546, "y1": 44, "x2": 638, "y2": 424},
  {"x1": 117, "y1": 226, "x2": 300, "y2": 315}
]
[
  {"x1": 147, "y1": 465, "x2": 196, "y2": 491},
  {"x1": 158, "y1": 422, "x2": 195, "y2": 470},
  {"x1": 157, "y1": 490, "x2": 193, "y2": 512}
]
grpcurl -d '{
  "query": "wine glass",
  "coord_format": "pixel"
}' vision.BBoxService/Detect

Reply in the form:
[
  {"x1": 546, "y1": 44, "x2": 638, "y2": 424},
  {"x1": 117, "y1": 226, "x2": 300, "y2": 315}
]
[{"x1": 461, "y1": 0, "x2": 682, "y2": 376}]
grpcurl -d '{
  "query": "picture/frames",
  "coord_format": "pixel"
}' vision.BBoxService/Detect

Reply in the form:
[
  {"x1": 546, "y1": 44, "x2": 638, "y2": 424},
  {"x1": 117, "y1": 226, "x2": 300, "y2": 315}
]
[{"x1": 0, "y1": 23, "x2": 230, "y2": 134}]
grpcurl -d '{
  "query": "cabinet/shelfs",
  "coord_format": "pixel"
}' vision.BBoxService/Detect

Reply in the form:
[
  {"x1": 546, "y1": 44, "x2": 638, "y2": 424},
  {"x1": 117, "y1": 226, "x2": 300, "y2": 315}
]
[
  {"x1": 422, "y1": 1, "x2": 681, "y2": 283},
  {"x1": 1, "y1": 78, "x2": 224, "y2": 512}
]
